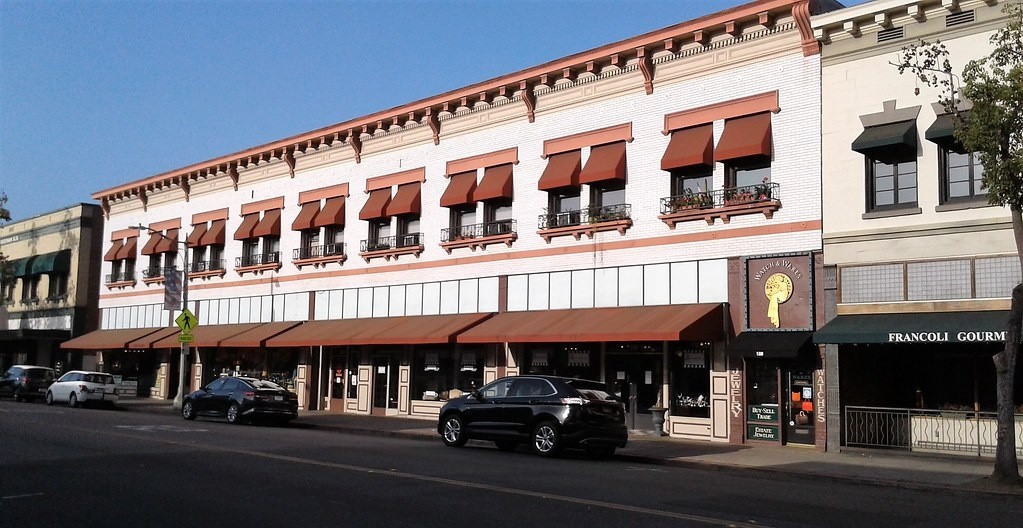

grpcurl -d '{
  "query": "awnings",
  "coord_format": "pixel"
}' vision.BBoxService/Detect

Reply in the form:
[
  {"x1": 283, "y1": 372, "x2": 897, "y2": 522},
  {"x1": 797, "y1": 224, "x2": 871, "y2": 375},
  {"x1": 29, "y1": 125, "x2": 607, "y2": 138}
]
[
  {"x1": 538, "y1": 151, "x2": 580, "y2": 191},
  {"x1": 724, "y1": 332, "x2": 812, "y2": 358},
  {"x1": 60, "y1": 303, "x2": 723, "y2": 349},
  {"x1": 473, "y1": 163, "x2": 512, "y2": 202},
  {"x1": 440, "y1": 171, "x2": 475, "y2": 207},
  {"x1": 0, "y1": 248, "x2": 69, "y2": 280},
  {"x1": 105, "y1": 237, "x2": 136, "y2": 260},
  {"x1": 715, "y1": 113, "x2": 771, "y2": 162},
  {"x1": 292, "y1": 197, "x2": 344, "y2": 231},
  {"x1": 386, "y1": 182, "x2": 420, "y2": 216},
  {"x1": 187, "y1": 221, "x2": 225, "y2": 248},
  {"x1": 579, "y1": 142, "x2": 626, "y2": 187},
  {"x1": 359, "y1": 189, "x2": 391, "y2": 220},
  {"x1": 925, "y1": 110, "x2": 969, "y2": 144},
  {"x1": 141, "y1": 230, "x2": 177, "y2": 254},
  {"x1": 851, "y1": 120, "x2": 917, "y2": 158},
  {"x1": 813, "y1": 310, "x2": 1023, "y2": 342},
  {"x1": 234, "y1": 209, "x2": 280, "y2": 239},
  {"x1": 661, "y1": 125, "x2": 713, "y2": 173}
]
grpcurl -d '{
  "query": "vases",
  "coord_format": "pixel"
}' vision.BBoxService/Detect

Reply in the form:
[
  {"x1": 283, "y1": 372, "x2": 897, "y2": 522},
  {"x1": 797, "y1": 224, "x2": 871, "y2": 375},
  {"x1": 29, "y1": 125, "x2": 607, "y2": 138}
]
[
  {"x1": 678, "y1": 205, "x2": 701, "y2": 209},
  {"x1": 724, "y1": 193, "x2": 769, "y2": 205}
]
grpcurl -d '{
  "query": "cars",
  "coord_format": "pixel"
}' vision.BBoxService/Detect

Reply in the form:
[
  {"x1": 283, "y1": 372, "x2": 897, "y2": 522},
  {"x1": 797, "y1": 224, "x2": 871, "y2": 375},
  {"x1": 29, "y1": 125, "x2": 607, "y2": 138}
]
[
  {"x1": 180, "y1": 376, "x2": 300, "y2": 427},
  {"x1": 45, "y1": 369, "x2": 119, "y2": 407}
]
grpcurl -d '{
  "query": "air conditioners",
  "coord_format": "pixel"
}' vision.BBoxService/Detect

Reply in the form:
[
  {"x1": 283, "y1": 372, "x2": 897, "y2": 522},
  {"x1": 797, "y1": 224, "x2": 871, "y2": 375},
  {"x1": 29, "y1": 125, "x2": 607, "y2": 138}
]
[
  {"x1": 557, "y1": 212, "x2": 571, "y2": 224},
  {"x1": 326, "y1": 244, "x2": 343, "y2": 255},
  {"x1": 267, "y1": 253, "x2": 279, "y2": 263},
  {"x1": 487, "y1": 224, "x2": 501, "y2": 234},
  {"x1": 403, "y1": 236, "x2": 415, "y2": 246}
]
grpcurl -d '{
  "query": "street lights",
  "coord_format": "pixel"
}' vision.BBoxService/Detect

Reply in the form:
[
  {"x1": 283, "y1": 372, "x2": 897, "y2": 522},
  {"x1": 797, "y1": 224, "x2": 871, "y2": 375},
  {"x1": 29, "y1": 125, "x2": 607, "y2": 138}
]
[{"x1": 128, "y1": 223, "x2": 190, "y2": 409}]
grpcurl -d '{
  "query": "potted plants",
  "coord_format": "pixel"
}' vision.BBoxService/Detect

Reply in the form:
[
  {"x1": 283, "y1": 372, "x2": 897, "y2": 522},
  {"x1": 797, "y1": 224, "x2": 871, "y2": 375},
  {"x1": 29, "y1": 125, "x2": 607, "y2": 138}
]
[{"x1": 647, "y1": 406, "x2": 669, "y2": 425}]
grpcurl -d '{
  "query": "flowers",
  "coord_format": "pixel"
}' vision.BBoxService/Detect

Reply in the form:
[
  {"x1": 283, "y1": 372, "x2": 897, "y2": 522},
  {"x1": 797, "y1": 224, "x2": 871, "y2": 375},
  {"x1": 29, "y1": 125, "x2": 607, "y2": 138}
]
[
  {"x1": 726, "y1": 177, "x2": 772, "y2": 197},
  {"x1": 669, "y1": 193, "x2": 703, "y2": 204}
]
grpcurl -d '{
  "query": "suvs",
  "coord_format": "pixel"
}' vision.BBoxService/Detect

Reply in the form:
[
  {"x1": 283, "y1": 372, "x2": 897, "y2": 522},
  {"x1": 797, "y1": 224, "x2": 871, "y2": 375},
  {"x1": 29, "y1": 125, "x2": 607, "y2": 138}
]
[
  {"x1": 437, "y1": 374, "x2": 629, "y2": 459},
  {"x1": 0, "y1": 364, "x2": 55, "y2": 402}
]
